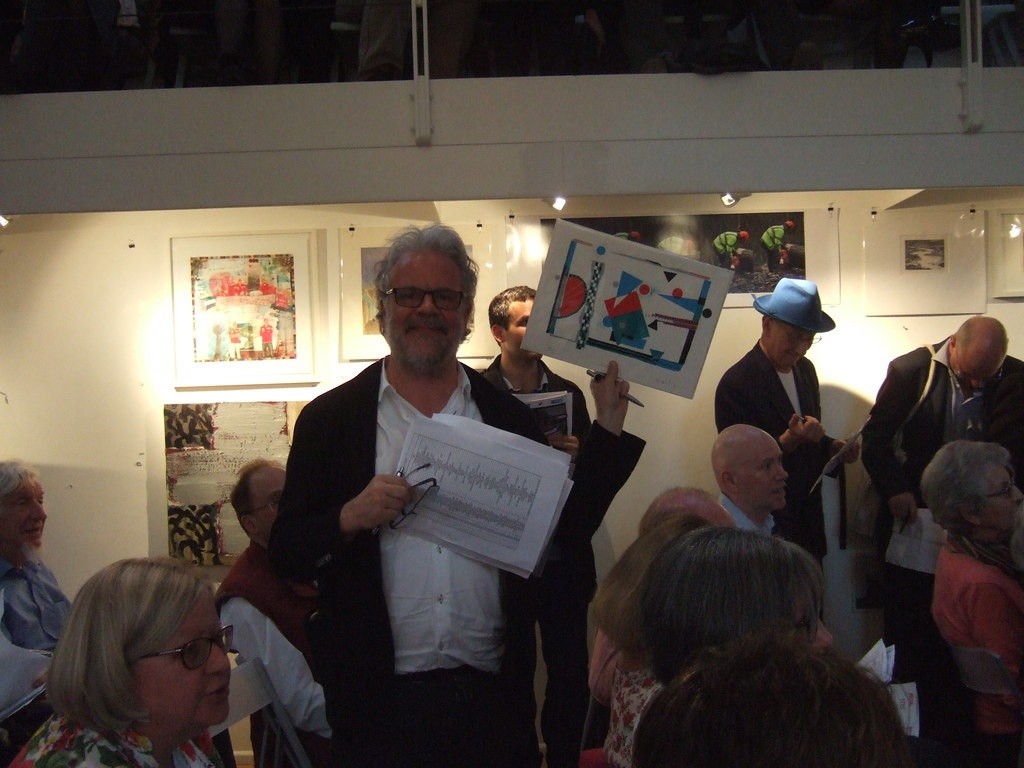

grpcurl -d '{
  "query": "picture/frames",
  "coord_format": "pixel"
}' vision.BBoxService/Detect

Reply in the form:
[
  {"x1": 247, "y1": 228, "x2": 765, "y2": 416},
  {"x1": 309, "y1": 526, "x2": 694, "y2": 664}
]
[
  {"x1": 994, "y1": 207, "x2": 1024, "y2": 298},
  {"x1": 170, "y1": 223, "x2": 509, "y2": 389}
]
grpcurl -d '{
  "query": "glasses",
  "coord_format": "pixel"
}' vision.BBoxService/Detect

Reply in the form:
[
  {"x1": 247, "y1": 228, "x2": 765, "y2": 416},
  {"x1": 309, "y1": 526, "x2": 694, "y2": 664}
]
[
  {"x1": 388, "y1": 463, "x2": 440, "y2": 529},
  {"x1": 126, "y1": 624, "x2": 235, "y2": 669},
  {"x1": 795, "y1": 603, "x2": 818, "y2": 643},
  {"x1": 244, "y1": 500, "x2": 281, "y2": 515},
  {"x1": 980, "y1": 475, "x2": 1016, "y2": 499},
  {"x1": 954, "y1": 347, "x2": 1002, "y2": 385},
  {"x1": 382, "y1": 287, "x2": 471, "y2": 310}
]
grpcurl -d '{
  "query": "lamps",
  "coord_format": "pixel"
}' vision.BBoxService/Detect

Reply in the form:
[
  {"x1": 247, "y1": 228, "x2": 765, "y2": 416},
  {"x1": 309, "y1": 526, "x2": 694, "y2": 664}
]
[
  {"x1": 720, "y1": 191, "x2": 752, "y2": 207},
  {"x1": 542, "y1": 197, "x2": 568, "y2": 212}
]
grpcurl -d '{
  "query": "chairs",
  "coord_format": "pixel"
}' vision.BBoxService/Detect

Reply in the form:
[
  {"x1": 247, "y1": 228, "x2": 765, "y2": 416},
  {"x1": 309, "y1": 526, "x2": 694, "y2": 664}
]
[
  {"x1": 206, "y1": 656, "x2": 313, "y2": 768},
  {"x1": 946, "y1": 642, "x2": 1024, "y2": 768}
]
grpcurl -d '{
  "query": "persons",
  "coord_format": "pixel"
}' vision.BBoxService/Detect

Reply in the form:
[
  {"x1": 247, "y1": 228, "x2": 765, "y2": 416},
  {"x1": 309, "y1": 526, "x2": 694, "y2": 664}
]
[
  {"x1": 714, "y1": 277, "x2": 860, "y2": 567},
  {"x1": 920, "y1": 441, "x2": 1024, "y2": 753},
  {"x1": 614, "y1": 231, "x2": 639, "y2": 240},
  {"x1": 0, "y1": 458, "x2": 74, "y2": 749},
  {"x1": 481, "y1": 285, "x2": 599, "y2": 767},
  {"x1": 712, "y1": 222, "x2": 796, "y2": 275},
  {"x1": 271, "y1": 225, "x2": 647, "y2": 768},
  {"x1": 213, "y1": 464, "x2": 333, "y2": 768},
  {"x1": 9, "y1": 556, "x2": 232, "y2": 768},
  {"x1": 579, "y1": 425, "x2": 914, "y2": 768},
  {"x1": 858, "y1": 318, "x2": 1023, "y2": 689}
]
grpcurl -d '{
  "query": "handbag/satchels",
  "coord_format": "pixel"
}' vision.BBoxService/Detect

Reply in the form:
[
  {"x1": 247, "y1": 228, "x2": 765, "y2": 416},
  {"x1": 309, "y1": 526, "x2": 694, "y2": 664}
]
[{"x1": 846, "y1": 443, "x2": 907, "y2": 548}]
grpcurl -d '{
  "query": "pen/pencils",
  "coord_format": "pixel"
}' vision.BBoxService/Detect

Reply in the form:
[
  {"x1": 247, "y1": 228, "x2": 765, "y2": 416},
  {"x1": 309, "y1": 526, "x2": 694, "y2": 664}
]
[
  {"x1": 372, "y1": 466, "x2": 405, "y2": 535},
  {"x1": 586, "y1": 370, "x2": 644, "y2": 408},
  {"x1": 798, "y1": 416, "x2": 806, "y2": 423},
  {"x1": 898, "y1": 511, "x2": 911, "y2": 535}
]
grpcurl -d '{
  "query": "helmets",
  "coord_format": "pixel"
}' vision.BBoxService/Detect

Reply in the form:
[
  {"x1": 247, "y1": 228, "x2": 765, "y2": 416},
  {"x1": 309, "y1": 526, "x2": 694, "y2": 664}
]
[
  {"x1": 740, "y1": 230, "x2": 748, "y2": 242},
  {"x1": 629, "y1": 231, "x2": 640, "y2": 241},
  {"x1": 786, "y1": 220, "x2": 795, "y2": 231}
]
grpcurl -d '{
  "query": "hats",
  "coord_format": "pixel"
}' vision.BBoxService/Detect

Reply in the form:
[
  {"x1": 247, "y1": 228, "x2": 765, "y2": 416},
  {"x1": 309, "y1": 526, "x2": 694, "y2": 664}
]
[{"x1": 753, "y1": 277, "x2": 836, "y2": 333}]
